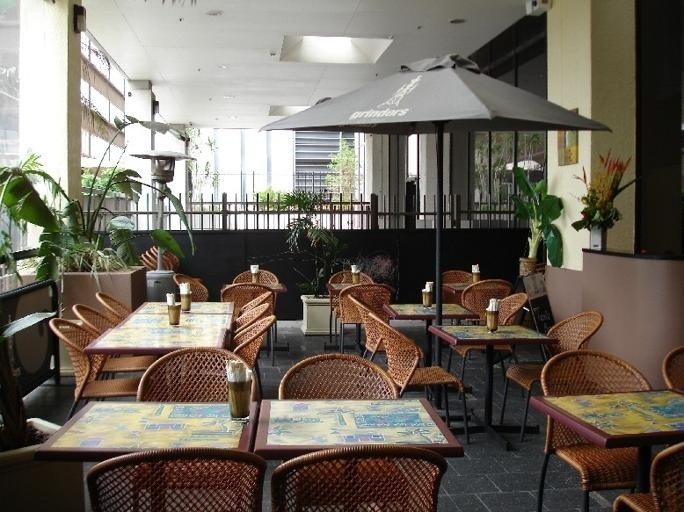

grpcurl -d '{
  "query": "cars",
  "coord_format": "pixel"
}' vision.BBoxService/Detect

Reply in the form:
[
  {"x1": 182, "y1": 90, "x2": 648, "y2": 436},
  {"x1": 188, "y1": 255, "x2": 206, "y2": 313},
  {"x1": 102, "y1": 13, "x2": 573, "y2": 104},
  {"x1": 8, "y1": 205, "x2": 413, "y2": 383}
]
[
  {"x1": 251, "y1": 272, "x2": 260, "y2": 284},
  {"x1": 422, "y1": 292, "x2": 432, "y2": 308},
  {"x1": 472, "y1": 272, "x2": 480, "y2": 283},
  {"x1": 486, "y1": 311, "x2": 498, "y2": 332},
  {"x1": 227, "y1": 379, "x2": 251, "y2": 422},
  {"x1": 181, "y1": 293, "x2": 191, "y2": 311},
  {"x1": 351, "y1": 272, "x2": 360, "y2": 285},
  {"x1": 168, "y1": 304, "x2": 181, "y2": 325}
]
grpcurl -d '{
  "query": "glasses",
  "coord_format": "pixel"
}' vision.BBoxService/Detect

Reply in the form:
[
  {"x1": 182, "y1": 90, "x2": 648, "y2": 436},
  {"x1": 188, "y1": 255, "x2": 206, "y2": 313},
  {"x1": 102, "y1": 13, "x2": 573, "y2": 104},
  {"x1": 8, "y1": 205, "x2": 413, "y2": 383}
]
[{"x1": 130, "y1": 152, "x2": 196, "y2": 302}]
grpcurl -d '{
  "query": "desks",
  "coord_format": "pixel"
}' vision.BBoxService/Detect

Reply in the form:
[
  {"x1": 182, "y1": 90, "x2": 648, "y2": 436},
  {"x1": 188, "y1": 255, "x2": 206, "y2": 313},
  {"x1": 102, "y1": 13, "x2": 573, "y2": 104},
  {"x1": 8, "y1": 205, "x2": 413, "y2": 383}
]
[
  {"x1": 129, "y1": 150, "x2": 198, "y2": 302},
  {"x1": 277, "y1": 193, "x2": 282, "y2": 196}
]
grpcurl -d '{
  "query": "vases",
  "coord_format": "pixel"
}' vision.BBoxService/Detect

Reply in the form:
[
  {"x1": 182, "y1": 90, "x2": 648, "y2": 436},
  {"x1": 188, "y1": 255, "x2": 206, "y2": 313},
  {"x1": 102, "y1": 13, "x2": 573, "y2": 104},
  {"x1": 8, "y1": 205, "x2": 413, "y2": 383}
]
[{"x1": 571, "y1": 149, "x2": 635, "y2": 231}]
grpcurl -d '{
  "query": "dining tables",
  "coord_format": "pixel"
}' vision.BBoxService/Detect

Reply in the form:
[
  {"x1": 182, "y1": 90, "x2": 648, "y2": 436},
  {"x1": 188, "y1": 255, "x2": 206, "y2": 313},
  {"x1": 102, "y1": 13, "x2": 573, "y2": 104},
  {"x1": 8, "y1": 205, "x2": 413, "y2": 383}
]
[
  {"x1": 0, "y1": 113, "x2": 191, "y2": 318},
  {"x1": 509, "y1": 167, "x2": 563, "y2": 275},
  {"x1": 280, "y1": 189, "x2": 352, "y2": 336}
]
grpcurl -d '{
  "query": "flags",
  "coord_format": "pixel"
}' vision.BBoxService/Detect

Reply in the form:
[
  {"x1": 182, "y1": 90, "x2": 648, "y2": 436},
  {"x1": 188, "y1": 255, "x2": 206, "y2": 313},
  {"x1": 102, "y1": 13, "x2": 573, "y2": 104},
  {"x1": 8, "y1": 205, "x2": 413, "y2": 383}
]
[{"x1": 590, "y1": 224, "x2": 607, "y2": 250}]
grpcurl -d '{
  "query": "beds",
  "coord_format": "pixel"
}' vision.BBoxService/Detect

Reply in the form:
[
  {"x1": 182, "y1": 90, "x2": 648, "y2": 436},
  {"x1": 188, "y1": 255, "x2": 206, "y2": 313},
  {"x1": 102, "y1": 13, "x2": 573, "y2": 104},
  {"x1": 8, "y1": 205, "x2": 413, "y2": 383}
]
[{"x1": 259, "y1": 53, "x2": 614, "y2": 422}]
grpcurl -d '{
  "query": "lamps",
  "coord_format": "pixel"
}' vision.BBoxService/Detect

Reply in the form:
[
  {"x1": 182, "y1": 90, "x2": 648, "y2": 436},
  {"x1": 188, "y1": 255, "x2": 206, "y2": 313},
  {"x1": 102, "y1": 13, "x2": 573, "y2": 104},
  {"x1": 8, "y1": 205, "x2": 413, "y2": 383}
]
[
  {"x1": 537, "y1": 350, "x2": 655, "y2": 512},
  {"x1": 441, "y1": 268, "x2": 473, "y2": 282},
  {"x1": 72, "y1": 303, "x2": 157, "y2": 401},
  {"x1": 278, "y1": 354, "x2": 399, "y2": 400},
  {"x1": 221, "y1": 283, "x2": 279, "y2": 366},
  {"x1": 96, "y1": 291, "x2": 132, "y2": 324},
  {"x1": 239, "y1": 290, "x2": 272, "y2": 313},
  {"x1": 661, "y1": 347, "x2": 684, "y2": 392},
  {"x1": 445, "y1": 293, "x2": 528, "y2": 401},
  {"x1": 612, "y1": 442, "x2": 684, "y2": 512},
  {"x1": 230, "y1": 303, "x2": 270, "y2": 334},
  {"x1": 368, "y1": 312, "x2": 471, "y2": 445},
  {"x1": 501, "y1": 310, "x2": 605, "y2": 443},
  {"x1": 338, "y1": 283, "x2": 394, "y2": 353},
  {"x1": 49, "y1": 318, "x2": 137, "y2": 422},
  {"x1": 87, "y1": 448, "x2": 267, "y2": 511},
  {"x1": 230, "y1": 315, "x2": 277, "y2": 399},
  {"x1": 460, "y1": 279, "x2": 512, "y2": 324},
  {"x1": 140, "y1": 244, "x2": 179, "y2": 271},
  {"x1": 328, "y1": 271, "x2": 374, "y2": 344},
  {"x1": 135, "y1": 347, "x2": 256, "y2": 402},
  {"x1": 234, "y1": 269, "x2": 278, "y2": 284},
  {"x1": 173, "y1": 273, "x2": 210, "y2": 301},
  {"x1": 270, "y1": 445, "x2": 448, "y2": 511}
]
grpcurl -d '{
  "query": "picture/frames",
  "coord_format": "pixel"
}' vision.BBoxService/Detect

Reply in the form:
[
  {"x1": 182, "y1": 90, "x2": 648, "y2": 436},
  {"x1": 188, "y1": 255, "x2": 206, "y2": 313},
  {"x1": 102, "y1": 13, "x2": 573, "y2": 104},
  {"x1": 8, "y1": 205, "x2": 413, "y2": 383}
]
[{"x1": 546, "y1": 248, "x2": 684, "y2": 388}]
